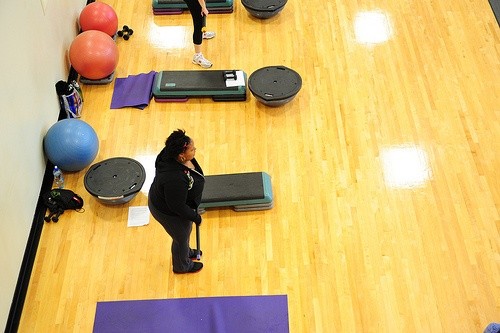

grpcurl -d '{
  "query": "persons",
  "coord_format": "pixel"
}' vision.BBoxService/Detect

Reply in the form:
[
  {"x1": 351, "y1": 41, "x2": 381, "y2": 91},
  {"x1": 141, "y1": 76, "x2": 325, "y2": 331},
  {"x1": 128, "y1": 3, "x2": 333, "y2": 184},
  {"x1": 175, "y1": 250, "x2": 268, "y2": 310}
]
[
  {"x1": 148, "y1": 129, "x2": 206, "y2": 274},
  {"x1": 182, "y1": 0, "x2": 219, "y2": 69}
]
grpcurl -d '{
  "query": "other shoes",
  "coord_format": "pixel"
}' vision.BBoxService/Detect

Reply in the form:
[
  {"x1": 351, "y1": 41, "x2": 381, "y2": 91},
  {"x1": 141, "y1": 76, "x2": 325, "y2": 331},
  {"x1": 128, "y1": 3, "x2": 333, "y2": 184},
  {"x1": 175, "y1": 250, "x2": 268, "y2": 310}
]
[
  {"x1": 190, "y1": 249, "x2": 202, "y2": 259},
  {"x1": 188, "y1": 262, "x2": 203, "y2": 273}
]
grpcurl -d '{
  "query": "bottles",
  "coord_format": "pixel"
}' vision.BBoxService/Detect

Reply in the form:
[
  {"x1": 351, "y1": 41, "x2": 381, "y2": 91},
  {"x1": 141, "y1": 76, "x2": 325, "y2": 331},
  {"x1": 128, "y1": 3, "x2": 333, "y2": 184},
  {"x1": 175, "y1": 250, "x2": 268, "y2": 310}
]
[
  {"x1": 58, "y1": 175, "x2": 64, "y2": 189},
  {"x1": 53, "y1": 166, "x2": 62, "y2": 184}
]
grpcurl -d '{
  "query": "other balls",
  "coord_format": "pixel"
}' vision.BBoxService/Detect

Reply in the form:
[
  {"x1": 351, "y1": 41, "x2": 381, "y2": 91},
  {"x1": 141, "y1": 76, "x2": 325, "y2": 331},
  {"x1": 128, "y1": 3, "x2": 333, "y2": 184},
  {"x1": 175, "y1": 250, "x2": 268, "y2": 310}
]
[
  {"x1": 69, "y1": 30, "x2": 119, "y2": 80},
  {"x1": 44, "y1": 118, "x2": 99, "y2": 172},
  {"x1": 79, "y1": 2, "x2": 118, "y2": 37}
]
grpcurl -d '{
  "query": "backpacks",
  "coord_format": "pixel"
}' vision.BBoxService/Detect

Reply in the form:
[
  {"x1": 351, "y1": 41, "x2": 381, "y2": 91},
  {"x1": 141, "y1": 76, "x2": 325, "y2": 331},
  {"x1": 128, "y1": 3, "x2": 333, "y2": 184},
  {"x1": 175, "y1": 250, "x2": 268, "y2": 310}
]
[
  {"x1": 55, "y1": 81, "x2": 82, "y2": 119},
  {"x1": 48, "y1": 189, "x2": 84, "y2": 212}
]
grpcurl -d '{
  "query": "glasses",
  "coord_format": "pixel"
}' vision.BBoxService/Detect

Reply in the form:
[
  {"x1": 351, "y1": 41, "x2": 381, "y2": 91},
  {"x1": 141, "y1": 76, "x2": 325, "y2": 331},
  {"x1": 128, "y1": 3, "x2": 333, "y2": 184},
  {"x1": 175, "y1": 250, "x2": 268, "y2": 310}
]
[{"x1": 176, "y1": 136, "x2": 189, "y2": 155}]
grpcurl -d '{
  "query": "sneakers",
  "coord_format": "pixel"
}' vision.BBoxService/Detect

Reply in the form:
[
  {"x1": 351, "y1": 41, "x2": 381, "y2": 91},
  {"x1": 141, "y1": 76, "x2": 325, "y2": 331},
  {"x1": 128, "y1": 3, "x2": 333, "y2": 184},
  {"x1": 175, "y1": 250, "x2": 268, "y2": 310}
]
[
  {"x1": 202, "y1": 32, "x2": 216, "y2": 38},
  {"x1": 192, "y1": 53, "x2": 213, "y2": 69}
]
